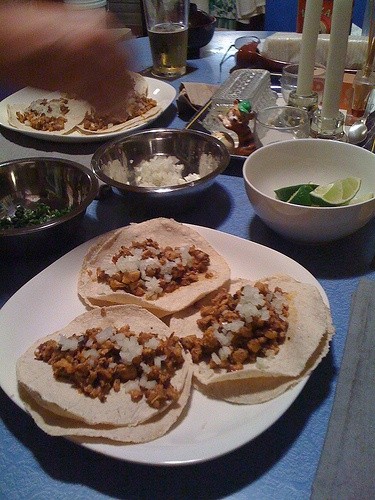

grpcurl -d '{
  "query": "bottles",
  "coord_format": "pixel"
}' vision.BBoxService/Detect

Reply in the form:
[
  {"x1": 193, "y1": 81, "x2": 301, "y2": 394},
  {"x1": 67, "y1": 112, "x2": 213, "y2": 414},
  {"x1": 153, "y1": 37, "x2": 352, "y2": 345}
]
[{"x1": 345, "y1": 36, "x2": 375, "y2": 125}]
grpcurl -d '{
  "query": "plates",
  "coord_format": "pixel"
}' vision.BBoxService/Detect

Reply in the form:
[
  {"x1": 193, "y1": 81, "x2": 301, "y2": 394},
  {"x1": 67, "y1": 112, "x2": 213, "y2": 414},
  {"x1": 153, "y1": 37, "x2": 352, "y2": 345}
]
[
  {"x1": 0, "y1": 223, "x2": 331, "y2": 468},
  {"x1": 0, "y1": 76, "x2": 177, "y2": 143},
  {"x1": 184, "y1": 74, "x2": 298, "y2": 160}
]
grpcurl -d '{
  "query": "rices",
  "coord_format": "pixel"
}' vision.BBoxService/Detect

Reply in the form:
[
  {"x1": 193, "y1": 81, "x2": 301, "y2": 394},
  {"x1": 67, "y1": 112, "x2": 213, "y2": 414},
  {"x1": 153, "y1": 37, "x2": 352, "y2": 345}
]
[{"x1": 135, "y1": 153, "x2": 200, "y2": 187}]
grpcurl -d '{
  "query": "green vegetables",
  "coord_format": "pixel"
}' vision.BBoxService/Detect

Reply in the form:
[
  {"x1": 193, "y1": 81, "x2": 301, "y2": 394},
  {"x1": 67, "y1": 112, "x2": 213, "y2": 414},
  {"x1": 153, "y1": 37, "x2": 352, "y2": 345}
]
[{"x1": 0, "y1": 202, "x2": 76, "y2": 230}]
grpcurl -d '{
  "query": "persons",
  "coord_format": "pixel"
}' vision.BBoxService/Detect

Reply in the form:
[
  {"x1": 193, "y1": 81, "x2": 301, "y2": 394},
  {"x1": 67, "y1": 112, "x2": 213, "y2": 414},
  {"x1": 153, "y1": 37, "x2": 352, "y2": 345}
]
[{"x1": 0, "y1": 0, "x2": 133, "y2": 112}]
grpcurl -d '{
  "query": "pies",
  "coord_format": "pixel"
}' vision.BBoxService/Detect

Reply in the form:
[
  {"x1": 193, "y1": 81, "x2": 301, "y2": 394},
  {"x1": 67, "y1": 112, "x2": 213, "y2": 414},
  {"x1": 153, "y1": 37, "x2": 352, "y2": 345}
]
[
  {"x1": 15, "y1": 217, "x2": 333, "y2": 444},
  {"x1": 7, "y1": 70, "x2": 162, "y2": 134}
]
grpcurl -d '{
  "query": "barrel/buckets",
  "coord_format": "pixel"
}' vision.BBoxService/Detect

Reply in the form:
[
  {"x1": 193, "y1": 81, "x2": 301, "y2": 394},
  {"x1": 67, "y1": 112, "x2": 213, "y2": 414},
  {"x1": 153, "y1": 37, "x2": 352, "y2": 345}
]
[{"x1": 64, "y1": 0, "x2": 110, "y2": 30}]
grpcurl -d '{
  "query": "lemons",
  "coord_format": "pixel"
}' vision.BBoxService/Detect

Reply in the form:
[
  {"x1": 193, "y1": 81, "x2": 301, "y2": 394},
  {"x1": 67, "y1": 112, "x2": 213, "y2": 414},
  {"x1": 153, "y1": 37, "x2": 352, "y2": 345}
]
[{"x1": 273, "y1": 176, "x2": 362, "y2": 207}]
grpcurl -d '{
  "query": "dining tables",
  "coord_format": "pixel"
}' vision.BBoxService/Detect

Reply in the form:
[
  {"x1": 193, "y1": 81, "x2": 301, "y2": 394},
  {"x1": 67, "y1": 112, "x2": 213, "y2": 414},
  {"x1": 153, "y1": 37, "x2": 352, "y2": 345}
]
[{"x1": 0, "y1": 31, "x2": 375, "y2": 500}]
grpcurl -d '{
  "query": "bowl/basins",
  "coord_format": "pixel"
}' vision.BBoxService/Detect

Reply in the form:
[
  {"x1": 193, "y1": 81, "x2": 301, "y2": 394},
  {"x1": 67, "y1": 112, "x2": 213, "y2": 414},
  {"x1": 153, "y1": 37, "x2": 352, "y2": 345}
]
[
  {"x1": 242, "y1": 138, "x2": 375, "y2": 246},
  {"x1": 91, "y1": 128, "x2": 229, "y2": 208},
  {"x1": 187, "y1": 15, "x2": 216, "y2": 60},
  {"x1": 0, "y1": 157, "x2": 100, "y2": 245}
]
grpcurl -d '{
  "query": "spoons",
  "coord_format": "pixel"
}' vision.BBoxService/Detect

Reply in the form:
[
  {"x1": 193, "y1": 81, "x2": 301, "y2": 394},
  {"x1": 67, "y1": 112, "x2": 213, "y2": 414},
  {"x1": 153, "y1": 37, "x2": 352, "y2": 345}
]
[{"x1": 348, "y1": 88, "x2": 375, "y2": 144}]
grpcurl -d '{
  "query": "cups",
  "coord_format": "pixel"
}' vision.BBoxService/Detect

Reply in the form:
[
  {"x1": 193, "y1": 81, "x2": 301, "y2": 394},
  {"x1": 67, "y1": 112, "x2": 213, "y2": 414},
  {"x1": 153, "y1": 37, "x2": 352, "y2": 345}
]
[
  {"x1": 281, "y1": 62, "x2": 327, "y2": 105},
  {"x1": 143, "y1": 0, "x2": 190, "y2": 79},
  {"x1": 253, "y1": 106, "x2": 310, "y2": 149}
]
